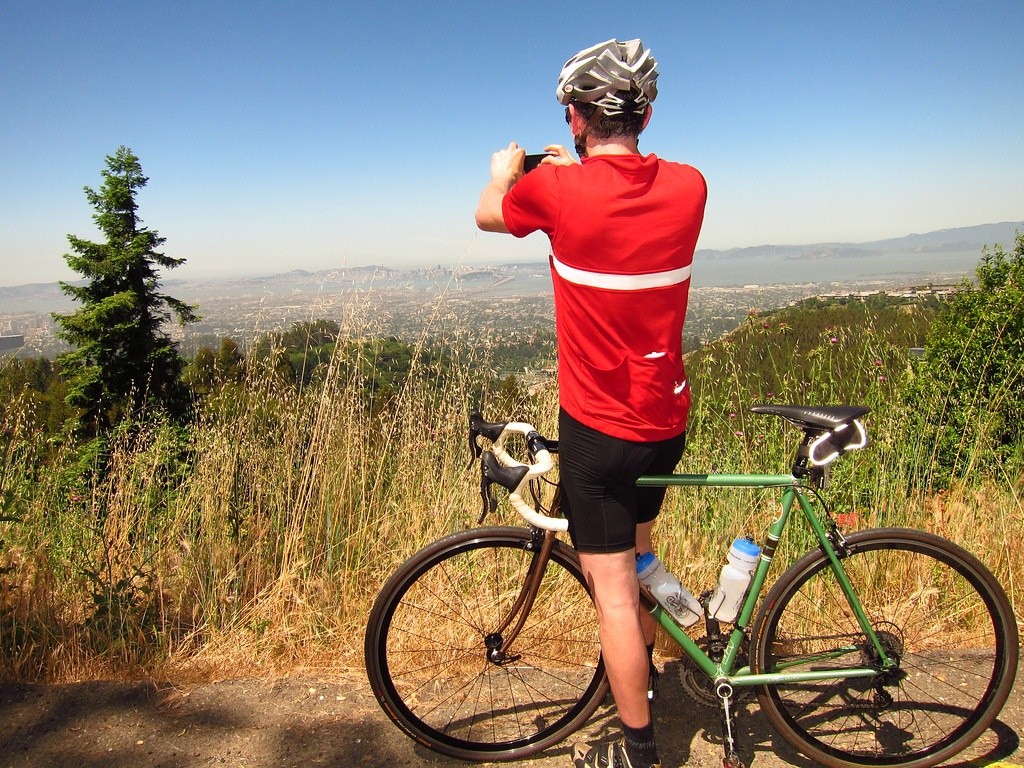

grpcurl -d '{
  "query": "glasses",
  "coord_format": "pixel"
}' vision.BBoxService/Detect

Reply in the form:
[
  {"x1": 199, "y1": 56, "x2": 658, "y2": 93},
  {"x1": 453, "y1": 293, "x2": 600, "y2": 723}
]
[{"x1": 565, "y1": 102, "x2": 580, "y2": 125}]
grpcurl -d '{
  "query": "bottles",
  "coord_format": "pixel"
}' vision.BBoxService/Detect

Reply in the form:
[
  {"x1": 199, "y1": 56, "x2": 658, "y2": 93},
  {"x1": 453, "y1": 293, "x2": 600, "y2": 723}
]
[
  {"x1": 710, "y1": 534, "x2": 760, "y2": 624},
  {"x1": 635, "y1": 553, "x2": 701, "y2": 627}
]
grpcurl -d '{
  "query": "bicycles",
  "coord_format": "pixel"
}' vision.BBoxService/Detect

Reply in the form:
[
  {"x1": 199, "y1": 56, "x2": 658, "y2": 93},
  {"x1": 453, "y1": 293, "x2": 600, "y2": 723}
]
[{"x1": 364, "y1": 401, "x2": 1019, "y2": 768}]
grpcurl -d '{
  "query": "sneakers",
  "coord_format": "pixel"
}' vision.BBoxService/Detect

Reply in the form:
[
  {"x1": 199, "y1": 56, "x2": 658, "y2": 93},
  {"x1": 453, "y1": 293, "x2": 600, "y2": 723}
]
[
  {"x1": 647, "y1": 662, "x2": 659, "y2": 702},
  {"x1": 570, "y1": 736, "x2": 662, "y2": 768}
]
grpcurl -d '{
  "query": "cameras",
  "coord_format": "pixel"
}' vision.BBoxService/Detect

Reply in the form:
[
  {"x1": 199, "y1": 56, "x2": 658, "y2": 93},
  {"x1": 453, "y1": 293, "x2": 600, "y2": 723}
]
[{"x1": 523, "y1": 153, "x2": 556, "y2": 175}]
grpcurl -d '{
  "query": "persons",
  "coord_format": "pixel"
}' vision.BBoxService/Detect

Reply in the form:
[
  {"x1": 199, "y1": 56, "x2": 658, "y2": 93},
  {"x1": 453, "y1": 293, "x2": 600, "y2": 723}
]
[{"x1": 475, "y1": 38, "x2": 708, "y2": 768}]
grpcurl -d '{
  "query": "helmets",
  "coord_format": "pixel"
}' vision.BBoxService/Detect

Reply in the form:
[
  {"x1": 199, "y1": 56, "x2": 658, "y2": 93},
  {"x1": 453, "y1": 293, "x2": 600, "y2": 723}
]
[{"x1": 556, "y1": 38, "x2": 660, "y2": 116}]
